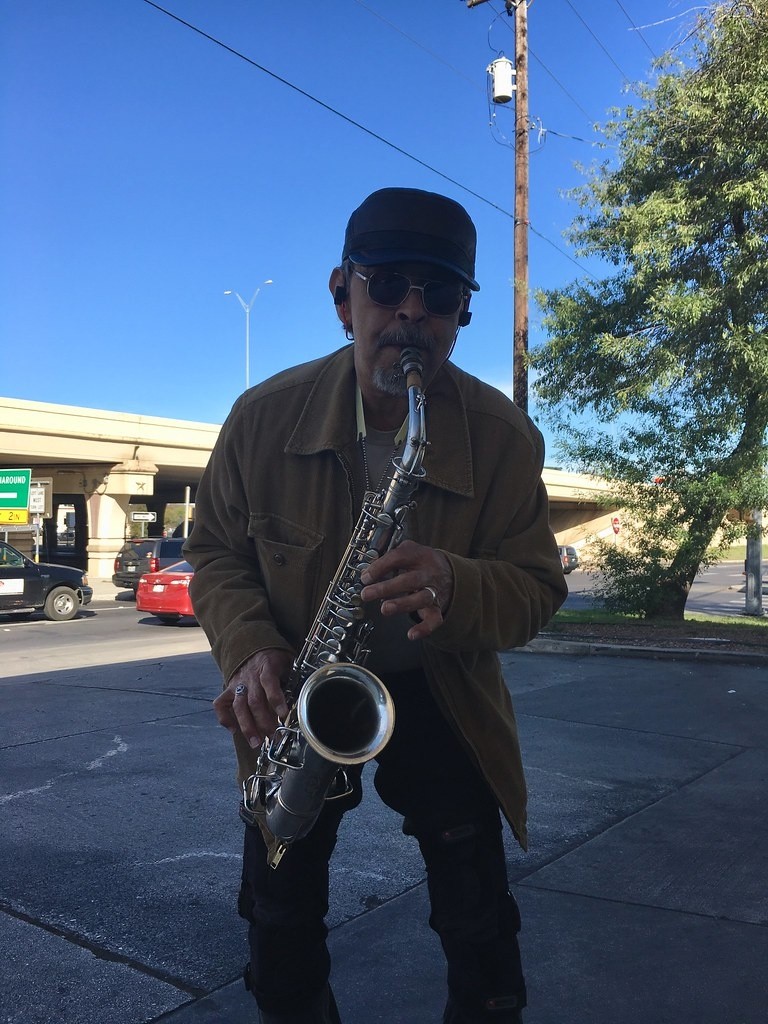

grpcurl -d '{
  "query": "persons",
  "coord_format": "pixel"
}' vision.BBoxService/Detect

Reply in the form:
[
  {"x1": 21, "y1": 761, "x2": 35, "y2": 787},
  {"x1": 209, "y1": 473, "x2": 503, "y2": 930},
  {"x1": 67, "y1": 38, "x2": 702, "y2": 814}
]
[{"x1": 183, "y1": 187, "x2": 568, "y2": 1024}]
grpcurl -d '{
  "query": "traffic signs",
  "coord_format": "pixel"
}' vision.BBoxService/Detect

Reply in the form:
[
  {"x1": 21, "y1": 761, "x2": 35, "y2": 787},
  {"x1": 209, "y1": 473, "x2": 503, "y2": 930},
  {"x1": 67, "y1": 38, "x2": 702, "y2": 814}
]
[{"x1": 1, "y1": 469, "x2": 33, "y2": 510}]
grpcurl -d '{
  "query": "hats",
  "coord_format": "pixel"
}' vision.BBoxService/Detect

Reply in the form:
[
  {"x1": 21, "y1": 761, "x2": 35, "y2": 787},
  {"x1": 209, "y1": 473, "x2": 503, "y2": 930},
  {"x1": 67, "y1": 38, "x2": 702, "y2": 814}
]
[{"x1": 342, "y1": 188, "x2": 480, "y2": 291}]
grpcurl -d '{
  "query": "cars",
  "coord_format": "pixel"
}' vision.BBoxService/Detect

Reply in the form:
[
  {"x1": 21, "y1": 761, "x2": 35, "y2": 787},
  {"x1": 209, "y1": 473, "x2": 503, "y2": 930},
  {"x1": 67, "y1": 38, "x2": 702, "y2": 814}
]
[
  {"x1": 58, "y1": 531, "x2": 77, "y2": 549},
  {"x1": 0, "y1": 541, "x2": 93, "y2": 621},
  {"x1": 136, "y1": 560, "x2": 202, "y2": 625}
]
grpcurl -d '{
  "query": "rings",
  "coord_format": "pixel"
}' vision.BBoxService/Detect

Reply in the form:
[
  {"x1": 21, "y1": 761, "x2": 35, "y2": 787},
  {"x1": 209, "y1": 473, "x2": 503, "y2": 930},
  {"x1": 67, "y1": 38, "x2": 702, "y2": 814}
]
[
  {"x1": 424, "y1": 586, "x2": 436, "y2": 602},
  {"x1": 234, "y1": 683, "x2": 247, "y2": 697}
]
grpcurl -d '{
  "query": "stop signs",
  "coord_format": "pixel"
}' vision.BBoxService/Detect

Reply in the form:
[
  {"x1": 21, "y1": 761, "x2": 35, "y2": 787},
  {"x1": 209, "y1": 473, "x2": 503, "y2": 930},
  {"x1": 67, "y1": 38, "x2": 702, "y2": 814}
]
[{"x1": 613, "y1": 518, "x2": 620, "y2": 534}]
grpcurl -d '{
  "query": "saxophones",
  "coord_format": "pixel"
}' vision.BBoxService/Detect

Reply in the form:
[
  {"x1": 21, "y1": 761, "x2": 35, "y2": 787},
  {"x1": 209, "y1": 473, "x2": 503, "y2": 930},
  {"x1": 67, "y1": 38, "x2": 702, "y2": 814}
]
[{"x1": 237, "y1": 346, "x2": 433, "y2": 846}]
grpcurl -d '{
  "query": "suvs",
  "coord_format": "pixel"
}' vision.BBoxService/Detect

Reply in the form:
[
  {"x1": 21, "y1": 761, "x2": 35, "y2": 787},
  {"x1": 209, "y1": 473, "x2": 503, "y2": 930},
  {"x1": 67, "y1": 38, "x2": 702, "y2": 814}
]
[
  {"x1": 557, "y1": 545, "x2": 579, "y2": 574},
  {"x1": 112, "y1": 537, "x2": 188, "y2": 598}
]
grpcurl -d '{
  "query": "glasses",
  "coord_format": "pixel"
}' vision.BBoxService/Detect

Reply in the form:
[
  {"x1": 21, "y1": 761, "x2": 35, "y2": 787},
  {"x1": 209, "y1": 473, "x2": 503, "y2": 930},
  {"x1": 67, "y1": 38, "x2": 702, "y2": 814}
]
[{"x1": 351, "y1": 267, "x2": 468, "y2": 317}]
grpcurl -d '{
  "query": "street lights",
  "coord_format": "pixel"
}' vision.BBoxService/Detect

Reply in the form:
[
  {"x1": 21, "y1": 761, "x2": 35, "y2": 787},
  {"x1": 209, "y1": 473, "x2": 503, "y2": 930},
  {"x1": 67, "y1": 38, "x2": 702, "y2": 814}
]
[{"x1": 223, "y1": 280, "x2": 275, "y2": 389}]
